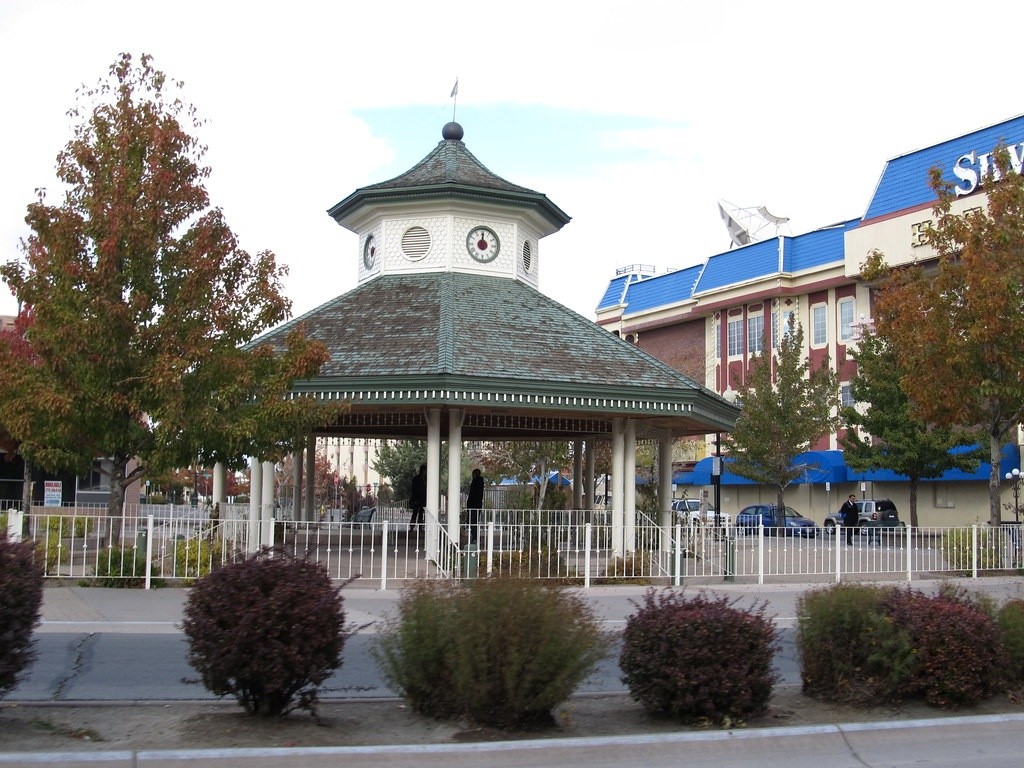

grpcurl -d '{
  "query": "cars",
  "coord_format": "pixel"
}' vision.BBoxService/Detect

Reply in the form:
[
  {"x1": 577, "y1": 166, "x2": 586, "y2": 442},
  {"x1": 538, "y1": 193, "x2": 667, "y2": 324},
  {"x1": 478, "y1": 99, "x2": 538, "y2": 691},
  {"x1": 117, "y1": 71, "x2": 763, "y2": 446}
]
[
  {"x1": 735, "y1": 505, "x2": 819, "y2": 539},
  {"x1": 592, "y1": 493, "x2": 612, "y2": 512}
]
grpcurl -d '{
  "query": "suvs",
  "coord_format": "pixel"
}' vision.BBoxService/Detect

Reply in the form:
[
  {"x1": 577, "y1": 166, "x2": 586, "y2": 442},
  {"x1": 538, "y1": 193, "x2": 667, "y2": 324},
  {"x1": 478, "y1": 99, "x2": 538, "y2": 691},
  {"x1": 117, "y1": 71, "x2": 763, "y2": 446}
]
[
  {"x1": 669, "y1": 499, "x2": 733, "y2": 535},
  {"x1": 824, "y1": 499, "x2": 899, "y2": 536}
]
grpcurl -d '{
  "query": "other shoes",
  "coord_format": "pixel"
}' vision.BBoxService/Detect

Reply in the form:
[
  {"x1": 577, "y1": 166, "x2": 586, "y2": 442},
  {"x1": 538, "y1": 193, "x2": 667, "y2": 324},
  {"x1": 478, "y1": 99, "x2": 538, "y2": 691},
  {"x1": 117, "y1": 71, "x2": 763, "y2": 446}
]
[
  {"x1": 471, "y1": 539, "x2": 480, "y2": 543},
  {"x1": 847, "y1": 541, "x2": 852, "y2": 545},
  {"x1": 409, "y1": 528, "x2": 424, "y2": 532}
]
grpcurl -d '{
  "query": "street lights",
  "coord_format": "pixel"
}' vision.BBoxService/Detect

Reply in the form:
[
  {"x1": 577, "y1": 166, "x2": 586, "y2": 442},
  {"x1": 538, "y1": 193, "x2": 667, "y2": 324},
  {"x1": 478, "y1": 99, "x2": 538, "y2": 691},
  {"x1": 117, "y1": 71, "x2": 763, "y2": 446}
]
[
  {"x1": 1005, "y1": 468, "x2": 1024, "y2": 521},
  {"x1": 186, "y1": 464, "x2": 205, "y2": 508}
]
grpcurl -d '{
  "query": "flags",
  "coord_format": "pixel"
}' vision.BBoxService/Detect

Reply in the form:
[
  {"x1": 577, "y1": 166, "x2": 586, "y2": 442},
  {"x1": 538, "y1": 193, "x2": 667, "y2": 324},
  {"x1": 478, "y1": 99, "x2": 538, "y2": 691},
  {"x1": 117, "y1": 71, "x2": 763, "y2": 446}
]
[{"x1": 450, "y1": 80, "x2": 458, "y2": 98}]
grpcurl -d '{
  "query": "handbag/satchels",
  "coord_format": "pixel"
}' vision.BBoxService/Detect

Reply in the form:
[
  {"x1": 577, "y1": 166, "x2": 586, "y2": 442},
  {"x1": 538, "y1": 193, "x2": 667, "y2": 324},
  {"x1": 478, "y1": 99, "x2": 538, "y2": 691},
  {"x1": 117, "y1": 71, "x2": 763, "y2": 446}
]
[{"x1": 839, "y1": 510, "x2": 847, "y2": 518}]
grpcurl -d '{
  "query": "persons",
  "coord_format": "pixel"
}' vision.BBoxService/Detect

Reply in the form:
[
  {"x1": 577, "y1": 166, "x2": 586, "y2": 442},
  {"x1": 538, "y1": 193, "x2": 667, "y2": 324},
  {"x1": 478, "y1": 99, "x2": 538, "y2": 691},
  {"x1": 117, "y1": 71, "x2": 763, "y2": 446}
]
[
  {"x1": 836, "y1": 495, "x2": 858, "y2": 547},
  {"x1": 407, "y1": 462, "x2": 427, "y2": 533},
  {"x1": 466, "y1": 469, "x2": 485, "y2": 545}
]
